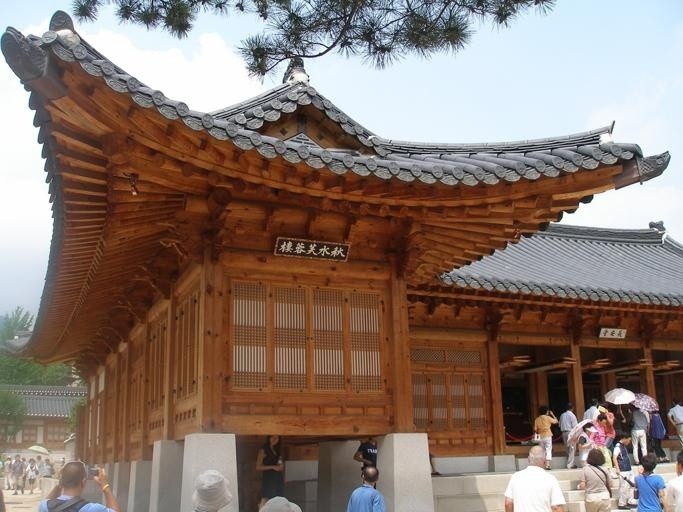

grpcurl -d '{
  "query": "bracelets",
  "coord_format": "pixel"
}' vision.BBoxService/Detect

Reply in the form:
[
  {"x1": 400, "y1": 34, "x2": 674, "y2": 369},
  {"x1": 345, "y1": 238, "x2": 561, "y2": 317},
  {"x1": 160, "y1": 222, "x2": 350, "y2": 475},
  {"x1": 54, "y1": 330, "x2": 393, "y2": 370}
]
[{"x1": 101, "y1": 484, "x2": 109, "y2": 493}]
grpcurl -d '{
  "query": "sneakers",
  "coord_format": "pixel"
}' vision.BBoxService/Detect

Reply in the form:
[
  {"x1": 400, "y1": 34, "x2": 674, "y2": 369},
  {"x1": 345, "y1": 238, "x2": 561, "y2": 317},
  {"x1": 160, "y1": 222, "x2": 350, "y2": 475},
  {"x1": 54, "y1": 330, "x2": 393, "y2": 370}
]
[{"x1": 618, "y1": 504, "x2": 636, "y2": 509}]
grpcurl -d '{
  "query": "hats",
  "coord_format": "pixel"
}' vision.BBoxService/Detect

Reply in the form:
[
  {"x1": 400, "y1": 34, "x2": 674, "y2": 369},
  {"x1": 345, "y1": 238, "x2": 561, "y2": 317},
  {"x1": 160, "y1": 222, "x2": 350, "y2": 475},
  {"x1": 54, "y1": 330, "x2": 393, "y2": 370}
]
[
  {"x1": 586, "y1": 426, "x2": 598, "y2": 433},
  {"x1": 193, "y1": 470, "x2": 231, "y2": 512}
]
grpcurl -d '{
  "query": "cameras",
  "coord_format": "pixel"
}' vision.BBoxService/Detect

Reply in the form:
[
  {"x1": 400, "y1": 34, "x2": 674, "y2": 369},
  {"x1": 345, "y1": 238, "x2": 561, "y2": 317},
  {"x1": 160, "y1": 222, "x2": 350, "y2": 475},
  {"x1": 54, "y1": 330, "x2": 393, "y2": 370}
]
[{"x1": 87, "y1": 467, "x2": 98, "y2": 480}]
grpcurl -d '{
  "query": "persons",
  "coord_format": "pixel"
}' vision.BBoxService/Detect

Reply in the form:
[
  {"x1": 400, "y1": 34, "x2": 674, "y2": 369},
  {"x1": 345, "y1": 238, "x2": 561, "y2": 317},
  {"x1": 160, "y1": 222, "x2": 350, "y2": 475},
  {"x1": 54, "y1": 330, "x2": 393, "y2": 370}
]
[
  {"x1": 253, "y1": 433, "x2": 285, "y2": 509},
  {"x1": 346, "y1": 464, "x2": 386, "y2": 511},
  {"x1": 558, "y1": 397, "x2": 682, "y2": 512},
  {"x1": 187, "y1": 471, "x2": 233, "y2": 511},
  {"x1": 38, "y1": 461, "x2": 121, "y2": 512},
  {"x1": 504, "y1": 445, "x2": 567, "y2": 511},
  {"x1": 0, "y1": 454, "x2": 67, "y2": 496},
  {"x1": 413, "y1": 421, "x2": 443, "y2": 477},
  {"x1": 532, "y1": 406, "x2": 558, "y2": 471},
  {"x1": 353, "y1": 436, "x2": 380, "y2": 489}
]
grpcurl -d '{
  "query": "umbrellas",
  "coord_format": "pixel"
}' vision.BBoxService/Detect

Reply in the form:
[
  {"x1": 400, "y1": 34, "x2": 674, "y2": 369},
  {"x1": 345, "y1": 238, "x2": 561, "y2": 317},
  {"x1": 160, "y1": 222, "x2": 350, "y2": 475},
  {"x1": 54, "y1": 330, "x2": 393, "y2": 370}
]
[
  {"x1": 27, "y1": 444, "x2": 48, "y2": 454},
  {"x1": 628, "y1": 393, "x2": 660, "y2": 413},
  {"x1": 605, "y1": 387, "x2": 635, "y2": 408}
]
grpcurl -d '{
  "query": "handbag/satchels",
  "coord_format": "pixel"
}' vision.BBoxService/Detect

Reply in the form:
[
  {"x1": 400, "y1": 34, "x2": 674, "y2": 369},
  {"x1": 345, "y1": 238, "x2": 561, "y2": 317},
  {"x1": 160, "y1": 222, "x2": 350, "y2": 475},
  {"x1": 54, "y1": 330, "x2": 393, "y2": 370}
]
[{"x1": 532, "y1": 433, "x2": 539, "y2": 444}]
